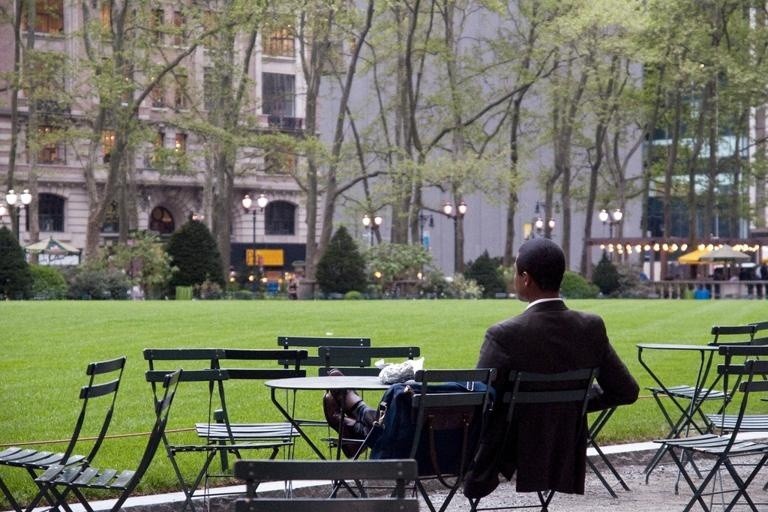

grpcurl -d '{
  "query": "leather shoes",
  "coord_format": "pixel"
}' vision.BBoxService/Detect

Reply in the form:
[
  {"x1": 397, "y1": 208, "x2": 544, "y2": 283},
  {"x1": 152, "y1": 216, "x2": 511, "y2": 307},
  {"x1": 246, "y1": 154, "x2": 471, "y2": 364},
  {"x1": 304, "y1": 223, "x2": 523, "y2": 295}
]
[
  {"x1": 329, "y1": 368, "x2": 365, "y2": 417},
  {"x1": 323, "y1": 396, "x2": 357, "y2": 457}
]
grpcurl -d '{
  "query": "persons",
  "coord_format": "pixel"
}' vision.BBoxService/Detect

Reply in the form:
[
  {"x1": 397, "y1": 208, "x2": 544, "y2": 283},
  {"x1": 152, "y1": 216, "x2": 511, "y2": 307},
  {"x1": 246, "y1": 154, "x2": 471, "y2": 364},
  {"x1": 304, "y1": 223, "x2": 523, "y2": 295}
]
[
  {"x1": 323, "y1": 235, "x2": 640, "y2": 499},
  {"x1": 287, "y1": 272, "x2": 300, "y2": 299}
]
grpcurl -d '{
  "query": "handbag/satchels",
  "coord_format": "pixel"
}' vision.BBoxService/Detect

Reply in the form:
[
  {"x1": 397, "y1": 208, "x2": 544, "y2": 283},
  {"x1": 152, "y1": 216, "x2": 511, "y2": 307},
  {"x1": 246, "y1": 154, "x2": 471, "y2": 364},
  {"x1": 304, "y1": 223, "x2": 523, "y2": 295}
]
[{"x1": 369, "y1": 382, "x2": 488, "y2": 475}]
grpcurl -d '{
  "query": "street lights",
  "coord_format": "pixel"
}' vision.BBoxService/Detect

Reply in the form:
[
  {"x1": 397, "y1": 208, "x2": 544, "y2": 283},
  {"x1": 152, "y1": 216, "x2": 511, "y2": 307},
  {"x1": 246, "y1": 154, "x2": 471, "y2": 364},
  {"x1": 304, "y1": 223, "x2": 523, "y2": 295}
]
[
  {"x1": 4, "y1": 188, "x2": 33, "y2": 242},
  {"x1": 444, "y1": 199, "x2": 468, "y2": 273},
  {"x1": 240, "y1": 191, "x2": 268, "y2": 276},
  {"x1": 361, "y1": 212, "x2": 383, "y2": 261},
  {"x1": 598, "y1": 208, "x2": 623, "y2": 263},
  {"x1": 535, "y1": 217, "x2": 556, "y2": 240}
]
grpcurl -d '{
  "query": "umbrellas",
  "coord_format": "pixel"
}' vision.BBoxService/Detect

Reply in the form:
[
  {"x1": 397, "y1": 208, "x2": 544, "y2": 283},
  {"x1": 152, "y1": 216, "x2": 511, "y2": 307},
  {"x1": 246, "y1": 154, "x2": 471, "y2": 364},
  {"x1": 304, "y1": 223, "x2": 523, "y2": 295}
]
[
  {"x1": 24, "y1": 235, "x2": 81, "y2": 257},
  {"x1": 699, "y1": 243, "x2": 751, "y2": 280},
  {"x1": 677, "y1": 248, "x2": 712, "y2": 277}
]
